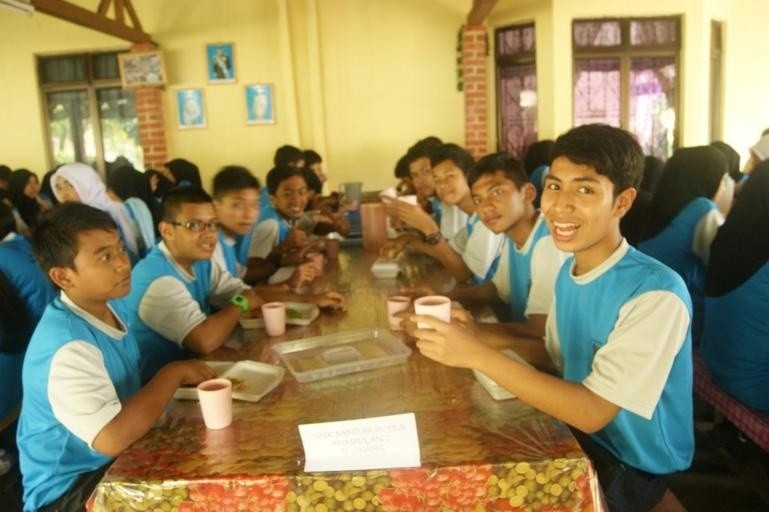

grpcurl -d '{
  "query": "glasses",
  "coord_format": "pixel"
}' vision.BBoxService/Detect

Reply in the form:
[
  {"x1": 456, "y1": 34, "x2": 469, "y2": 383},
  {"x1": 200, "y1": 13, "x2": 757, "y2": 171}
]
[{"x1": 168, "y1": 217, "x2": 220, "y2": 234}]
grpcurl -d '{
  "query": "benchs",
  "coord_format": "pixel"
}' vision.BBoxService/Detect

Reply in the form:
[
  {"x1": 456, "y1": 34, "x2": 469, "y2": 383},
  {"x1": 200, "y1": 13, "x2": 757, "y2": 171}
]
[{"x1": 692, "y1": 346, "x2": 769, "y2": 507}]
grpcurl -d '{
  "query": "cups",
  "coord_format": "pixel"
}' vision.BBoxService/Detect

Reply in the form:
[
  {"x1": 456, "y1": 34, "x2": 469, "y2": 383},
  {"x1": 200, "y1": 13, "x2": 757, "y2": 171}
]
[
  {"x1": 197, "y1": 379, "x2": 232, "y2": 430},
  {"x1": 327, "y1": 239, "x2": 338, "y2": 261},
  {"x1": 338, "y1": 181, "x2": 362, "y2": 207},
  {"x1": 308, "y1": 252, "x2": 324, "y2": 277},
  {"x1": 414, "y1": 295, "x2": 451, "y2": 329},
  {"x1": 398, "y1": 194, "x2": 416, "y2": 204},
  {"x1": 387, "y1": 296, "x2": 409, "y2": 332},
  {"x1": 261, "y1": 301, "x2": 286, "y2": 337},
  {"x1": 378, "y1": 187, "x2": 397, "y2": 205}
]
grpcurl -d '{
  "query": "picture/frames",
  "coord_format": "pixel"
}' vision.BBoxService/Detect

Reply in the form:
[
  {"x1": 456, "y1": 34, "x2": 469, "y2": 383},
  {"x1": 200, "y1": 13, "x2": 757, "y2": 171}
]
[
  {"x1": 118, "y1": 51, "x2": 168, "y2": 91},
  {"x1": 206, "y1": 41, "x2": 237, "y2": 83},
  {"x1": 244, "y1": 83, "x2": 274, "y2": 125},
  {"x1": 176, "y1": 87, "x2": 206, "y2": 131}
]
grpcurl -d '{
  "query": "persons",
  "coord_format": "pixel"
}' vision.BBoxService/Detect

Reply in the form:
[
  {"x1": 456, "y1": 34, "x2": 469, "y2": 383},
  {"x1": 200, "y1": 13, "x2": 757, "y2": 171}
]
[
  {"x1": 379, "y1": 124, "x2": 768, "y2": 511},
  {"x1": 0, "y1": 145, "x2": 352, "y2": 511}
]
